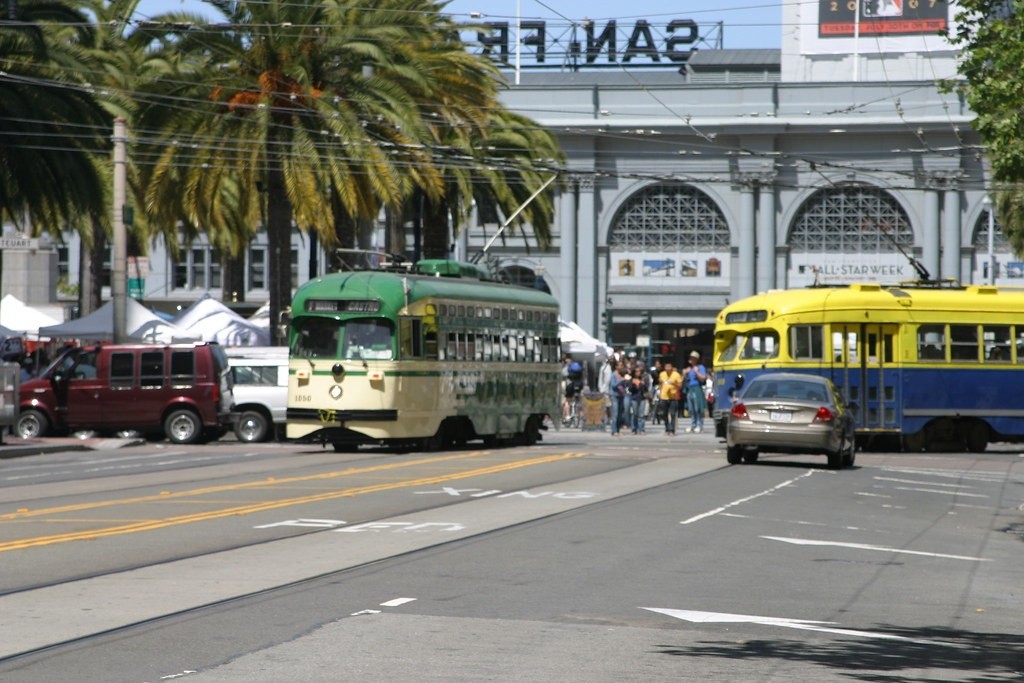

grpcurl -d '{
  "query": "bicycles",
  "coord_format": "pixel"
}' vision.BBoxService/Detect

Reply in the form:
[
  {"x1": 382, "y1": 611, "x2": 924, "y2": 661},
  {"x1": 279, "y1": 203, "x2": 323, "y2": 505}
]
[{"x1": 560, "y1": 387, "x2": 580, "y2": 428}]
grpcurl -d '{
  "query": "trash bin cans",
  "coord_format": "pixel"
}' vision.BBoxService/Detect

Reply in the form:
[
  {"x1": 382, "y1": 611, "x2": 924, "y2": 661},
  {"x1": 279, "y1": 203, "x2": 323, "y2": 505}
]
[{"x1": 581, "y1": 391, "x2": 606, "y2": 430}]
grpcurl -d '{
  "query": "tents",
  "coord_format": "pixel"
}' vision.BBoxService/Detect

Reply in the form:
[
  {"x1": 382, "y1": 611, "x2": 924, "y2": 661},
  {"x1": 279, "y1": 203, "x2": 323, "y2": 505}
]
[
  {"x1": 556, "y1": 317, "x2": 615, "y2": 391},
  {"x1": 0, "y1": 291, "x2": 291, "y2": 377}
]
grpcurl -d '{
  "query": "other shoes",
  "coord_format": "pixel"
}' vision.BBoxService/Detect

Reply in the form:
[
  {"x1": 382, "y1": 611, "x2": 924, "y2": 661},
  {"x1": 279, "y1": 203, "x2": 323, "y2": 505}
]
[
  {"x1": 685, "y1": 427, "x2": 693, "y2": 433},
  {"x1": 563, "y1": 415, "x2": 571, "y2": 423},
  {"x1": 694, "y1": 426, "x2": 702, "y2": 433}
]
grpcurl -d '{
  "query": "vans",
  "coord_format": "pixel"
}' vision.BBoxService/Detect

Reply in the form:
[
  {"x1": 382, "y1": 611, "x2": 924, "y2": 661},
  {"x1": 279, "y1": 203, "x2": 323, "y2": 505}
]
[
  {"x1": 221, "y1": 348, "x2": 291, "y2": 443},
  {"x1": 273, "y1": 259, "x2": 561, "y2": 452},
  {"x1": 14, "y1": 343, "x2": 236, "y2": 446}
]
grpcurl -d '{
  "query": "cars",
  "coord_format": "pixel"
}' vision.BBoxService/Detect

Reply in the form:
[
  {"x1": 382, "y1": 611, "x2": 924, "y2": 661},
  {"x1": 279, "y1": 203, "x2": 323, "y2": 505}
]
[{"x1": 727, "y1": 373, "x2": 856, "y2": 469}]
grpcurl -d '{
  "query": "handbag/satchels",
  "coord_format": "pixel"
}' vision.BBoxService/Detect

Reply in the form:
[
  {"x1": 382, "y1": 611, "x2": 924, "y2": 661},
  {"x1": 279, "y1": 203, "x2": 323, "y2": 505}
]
[{"x1": 628, "y1": 385, "x2": 636, "y2": 393}]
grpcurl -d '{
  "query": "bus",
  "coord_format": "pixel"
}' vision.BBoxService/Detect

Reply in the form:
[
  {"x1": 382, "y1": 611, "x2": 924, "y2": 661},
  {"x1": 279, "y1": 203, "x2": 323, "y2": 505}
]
[{"x1": 711, "y1": 279, "x2": 1024, "y2": 451}]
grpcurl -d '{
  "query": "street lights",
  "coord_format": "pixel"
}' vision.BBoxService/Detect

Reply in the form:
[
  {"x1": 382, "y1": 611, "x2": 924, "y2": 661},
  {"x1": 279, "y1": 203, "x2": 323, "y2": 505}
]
[{"x1": 983, "y1": 193, "x2": 995, "y2": 284}]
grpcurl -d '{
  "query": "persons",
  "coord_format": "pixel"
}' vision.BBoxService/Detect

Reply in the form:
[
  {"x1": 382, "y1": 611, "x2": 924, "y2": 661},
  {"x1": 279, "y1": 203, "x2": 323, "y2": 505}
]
[{"x1": 558, "y1": 349, "x2": 716, "y2": 439}]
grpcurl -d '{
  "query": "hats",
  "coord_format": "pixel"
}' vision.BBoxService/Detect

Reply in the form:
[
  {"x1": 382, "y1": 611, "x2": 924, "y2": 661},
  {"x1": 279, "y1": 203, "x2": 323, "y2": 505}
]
[
  {"x1": 628, "y1": 351, "x2": 637, "y2": 358},
  {"x1": 688, "y1": 350, "x2": 700, "y2": 359}
]
